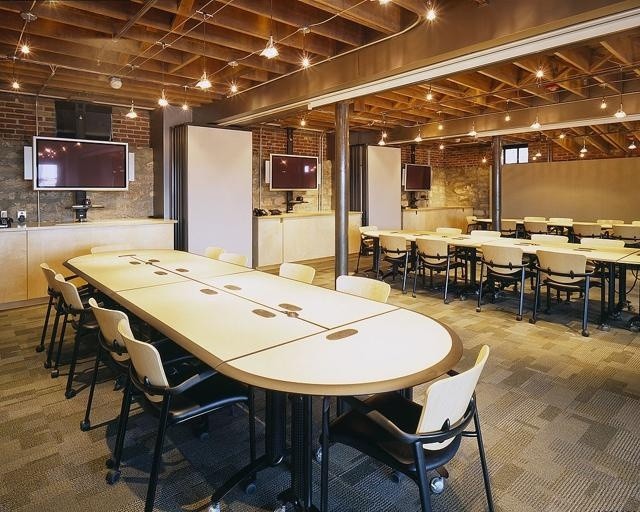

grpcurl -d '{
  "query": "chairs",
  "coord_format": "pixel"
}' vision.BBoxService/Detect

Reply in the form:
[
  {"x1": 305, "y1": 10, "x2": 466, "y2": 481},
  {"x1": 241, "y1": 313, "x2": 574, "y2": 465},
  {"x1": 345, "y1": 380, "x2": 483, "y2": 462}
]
[
  {"x1": 79, "y1": 296, "x2": 181, "y2": 468},
  {"x1": 276, "y1": 262, "x2": 315, "y2": 286},
  {"x1": 316, "y1": 344, "x2": 494, "y2": 512},
  {"x1": 204, "y1": 247, "x2": 224, "y2": 260},
  {"x1": 47, "y1": 273, "x2": 105, "y2": 399},
  {"x1": 356, "y1": 216, "x2": 640, "y2": 338},
  {"x1": 217, "y1": 251, "x2": 251, "y2": 270},
  {"x1": 35, "y1": 262, "x2": 103, "y2": 352},
  {"x1": 107, "y1": 317, "x2": 261, "y2": 511},
  {"x1": 334, "y1": 273, "x2": 390, "y2": 306}
]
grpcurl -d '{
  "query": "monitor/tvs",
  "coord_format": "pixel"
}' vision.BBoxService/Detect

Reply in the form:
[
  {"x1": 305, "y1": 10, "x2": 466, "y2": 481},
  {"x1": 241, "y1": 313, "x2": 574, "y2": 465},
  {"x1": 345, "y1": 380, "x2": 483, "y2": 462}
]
[
  {"x1": 404, "y1": 164, "x2": 432, "y2": 192},
  {"x1": 269, "y1": 153, "x2": 318, "y2": 191},
  {"x1": 33, "y1": 135, "x2": 129, "y2": 191}
]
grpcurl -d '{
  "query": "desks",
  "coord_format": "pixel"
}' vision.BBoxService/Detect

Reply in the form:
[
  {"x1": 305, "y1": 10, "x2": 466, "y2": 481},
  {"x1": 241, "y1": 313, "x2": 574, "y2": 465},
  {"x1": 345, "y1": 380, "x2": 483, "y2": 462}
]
[{"x1": 63, "y1": 246, "x2": 465, "y2": 512}]
